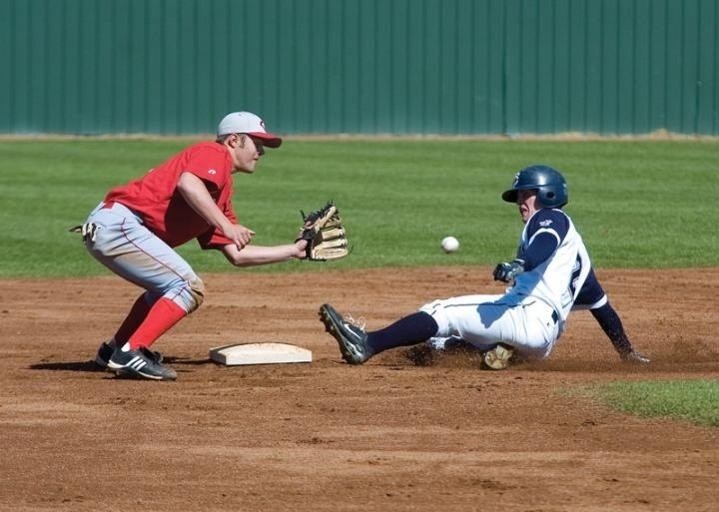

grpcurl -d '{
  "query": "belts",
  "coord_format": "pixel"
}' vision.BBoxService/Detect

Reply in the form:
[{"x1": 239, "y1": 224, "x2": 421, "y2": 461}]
[
  {"x1": 550, "y1": 311, "x2": 561, "y2": 340},
  {"x1": 98, "y1": 201, "x2": 115, "y2": 209}
]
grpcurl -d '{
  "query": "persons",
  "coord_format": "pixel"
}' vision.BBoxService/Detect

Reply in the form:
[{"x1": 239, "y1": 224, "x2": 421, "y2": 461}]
[
  {"x1": 318, "y1": 166, "x2": 652, "y2": 369},
  {"x1": 69, "y1": 110, "x2": 350, "y2": 381}
]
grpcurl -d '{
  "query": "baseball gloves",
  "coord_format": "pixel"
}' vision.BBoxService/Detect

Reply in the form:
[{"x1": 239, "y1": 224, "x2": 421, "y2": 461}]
[{"x1": 295, "y1": 200, "x2": 350, "y2": 261}]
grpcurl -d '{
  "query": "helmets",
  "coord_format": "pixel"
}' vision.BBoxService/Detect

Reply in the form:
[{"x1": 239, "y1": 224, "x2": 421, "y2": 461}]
[{"x1": 501, "y1": 164, "x2": 569, "y2": 208}]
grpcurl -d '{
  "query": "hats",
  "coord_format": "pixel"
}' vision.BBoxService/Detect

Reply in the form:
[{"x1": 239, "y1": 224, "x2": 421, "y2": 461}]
[{"x1": 217, "y1": 110, "x2": 283, "y2": 149}]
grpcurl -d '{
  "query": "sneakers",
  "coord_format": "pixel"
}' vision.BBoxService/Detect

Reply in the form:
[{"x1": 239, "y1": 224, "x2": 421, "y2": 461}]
[
  {"x1": 107, "y1": 347, "x2": 178, "y2": 380},
  {"x1": 481, "y1": 342, "x2": 515, "y2": 370},
  {"x1": 317, "y1": 303, "x2": 373, "y2": 365},
  {"x1": 94, "y1": 340, "x2": 163, "y2": 368}
]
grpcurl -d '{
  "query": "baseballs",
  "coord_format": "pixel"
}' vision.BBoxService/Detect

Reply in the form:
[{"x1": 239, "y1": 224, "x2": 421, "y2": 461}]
[{"x1": 440, "y1": 235, "x2": 458, "y2": 253}]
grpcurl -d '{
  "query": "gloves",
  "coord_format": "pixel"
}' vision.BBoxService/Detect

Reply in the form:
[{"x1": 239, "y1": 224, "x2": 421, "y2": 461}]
[
  {"x1": 620, "y1": 350, "x2": 650, "y2": 363},
  {"x1": 68, "y1": 222, "x2": 97, "y2": 243},
  {"x1": 492, "y1": 258, "x2": 526, "y2": 283}
]
